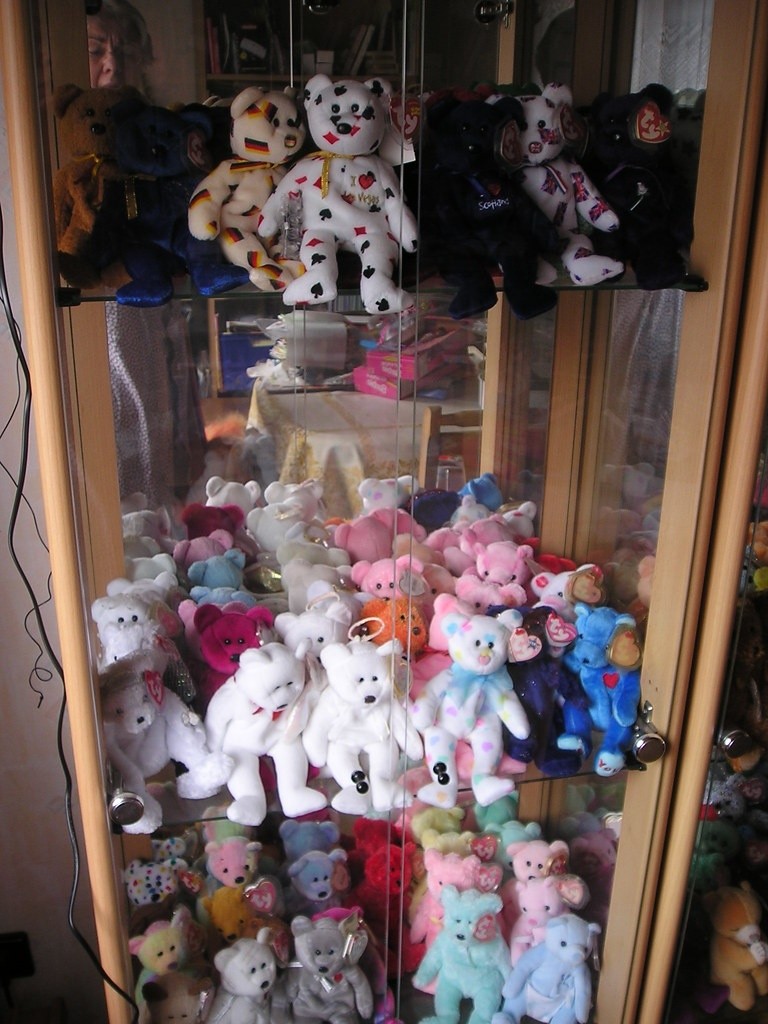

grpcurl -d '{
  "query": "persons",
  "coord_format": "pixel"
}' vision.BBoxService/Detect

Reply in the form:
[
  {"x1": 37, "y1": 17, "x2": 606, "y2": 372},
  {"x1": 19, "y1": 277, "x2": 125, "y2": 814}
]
[{"x1": 83, "y1": 0, "x2": 211, "y2": 512}]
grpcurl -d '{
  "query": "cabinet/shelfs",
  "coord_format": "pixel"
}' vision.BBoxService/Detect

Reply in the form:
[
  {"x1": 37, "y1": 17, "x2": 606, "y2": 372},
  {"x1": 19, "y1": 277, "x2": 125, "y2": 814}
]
[
  {"x1": 192, "y1": 0, "x2": 422, "y2": 398},
  {"x1": 0, "y1": 0, "x2": 768, "y2": 1024}
]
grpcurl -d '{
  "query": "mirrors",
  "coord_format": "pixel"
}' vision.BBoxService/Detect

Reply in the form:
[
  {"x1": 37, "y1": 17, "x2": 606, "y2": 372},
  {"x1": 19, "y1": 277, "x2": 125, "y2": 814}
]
[{"x1": 85, "y1": 0, "x2": 574, "y2": 1024}]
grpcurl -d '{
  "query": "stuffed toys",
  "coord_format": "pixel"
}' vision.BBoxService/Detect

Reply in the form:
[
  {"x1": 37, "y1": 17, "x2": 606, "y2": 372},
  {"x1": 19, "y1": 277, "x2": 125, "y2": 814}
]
[
  {"x1": 117, "y1": 474, "x2": 661, "y2": 566},
  {"x1": 98, "y1": 509, "x2": 768, "y2": 1024},
  {"x1": 48, "y1": 74, "x2": 711, "y2": 315}
]
